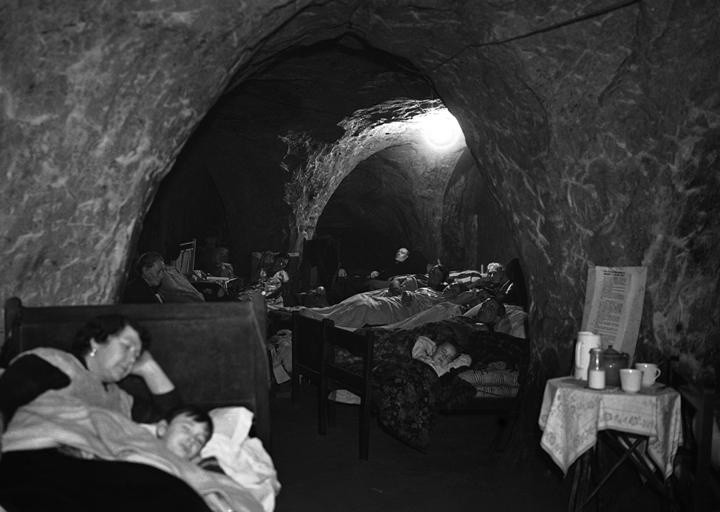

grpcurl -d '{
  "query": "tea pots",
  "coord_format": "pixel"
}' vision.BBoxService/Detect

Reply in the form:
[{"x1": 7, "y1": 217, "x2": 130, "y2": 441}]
[{"x1": 602, "y1": 345, "x2": 628, "y2": 386}]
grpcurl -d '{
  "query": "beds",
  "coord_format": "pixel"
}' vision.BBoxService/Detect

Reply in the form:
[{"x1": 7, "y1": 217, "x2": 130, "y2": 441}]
[
  {"x1": 1, "y1": 295, "x2": 277, "y2": 511},
  {"x1": 288, "y1": 276, "x2": 529, "y2": 461}
]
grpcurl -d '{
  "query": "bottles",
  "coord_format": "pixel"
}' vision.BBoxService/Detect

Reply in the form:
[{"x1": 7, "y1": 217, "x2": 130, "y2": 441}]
[
  {"x1": 574, "y1": 331, "x2": 602, "y2": 381},
  {"x1": 589, "y1": 348, "x2": 607, "y2": 389}
]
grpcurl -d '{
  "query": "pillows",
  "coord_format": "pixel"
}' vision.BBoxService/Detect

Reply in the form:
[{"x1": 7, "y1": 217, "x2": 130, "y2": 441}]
[{"x1": 140, "y1": 405, "x2": 255, "y2": 462}]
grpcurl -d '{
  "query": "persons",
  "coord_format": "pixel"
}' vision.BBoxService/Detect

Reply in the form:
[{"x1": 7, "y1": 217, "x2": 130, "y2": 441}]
[
  {"x1": 124, "y1": 251, "x2": 165, "y2": 303},
  {"x1": 1, "y1": 314, "x2": 212, "y2": 512},
  {"x1": 189, "y1": 242, "x2": 534, "y2": 376},
  {"x1": 154, "y1": 406, "x2": 226, "y2": 477}
]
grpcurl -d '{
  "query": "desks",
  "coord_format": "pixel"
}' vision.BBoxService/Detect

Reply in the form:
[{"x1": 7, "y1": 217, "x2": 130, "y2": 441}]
[{"x1": 548, "y1": 374, "x2": 680, "y2": 510}]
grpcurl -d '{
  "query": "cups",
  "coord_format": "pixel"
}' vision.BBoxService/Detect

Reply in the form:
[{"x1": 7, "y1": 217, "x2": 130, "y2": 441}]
[
  {"x1": 636, "y1": 363, "x2": 661, "y2": 387},
  {"x1": 620, "y1": 369, "x2": 643, "y2": 393}
]
